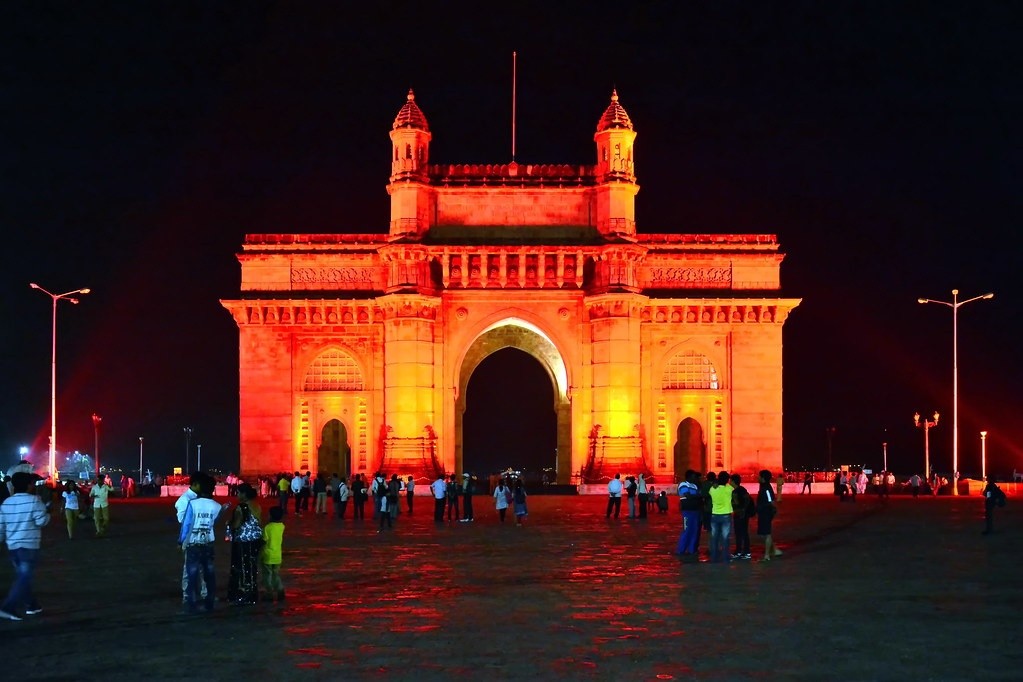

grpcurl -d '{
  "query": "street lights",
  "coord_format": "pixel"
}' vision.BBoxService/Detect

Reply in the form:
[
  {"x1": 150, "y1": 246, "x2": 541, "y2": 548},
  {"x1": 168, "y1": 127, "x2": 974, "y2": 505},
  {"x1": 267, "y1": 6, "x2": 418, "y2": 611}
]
[
  {"x1": 882, "y1": 442, "x2": 888, "y2": 471},
  {"x1": 19, "y1": 446, "x2": 28, "y2": 460},
  {"x1": 91, "y1": 412, "x2": 103, "y2": 484},
  {"x1": 29, "y1": 282, "x2": 90, "y2": 490},
  {"x1": 979, "y1": 431, "x2": 988, "y2": 492},
  {"x1": 914, "y1": 411, "x2": 941, "y2": 483},
  {"x1": 917, "y1": 289, "x2": 995, "y2": 496},
  {"x1": 138, "y1": 437, "x2": 144, "y2": 486},
  {"x1": 183, "y1": 426, "x2": 191, "y2": 475},
  {"x1": 196, "y1": 445, "x2": 201, "y2": 471}
]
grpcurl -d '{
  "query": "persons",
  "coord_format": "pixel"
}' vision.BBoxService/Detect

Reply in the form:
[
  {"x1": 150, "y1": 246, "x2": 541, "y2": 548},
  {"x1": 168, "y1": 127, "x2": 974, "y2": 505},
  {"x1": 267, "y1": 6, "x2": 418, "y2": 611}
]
[
  {"x1": 834, "y1": 468, "x2": 951, "y2": 503},
  {"x1": 512, "y1": 479, "x2": 528, "y2": 527},
  {"x1": 88, "y1": 475, "x2": 115, "y2": 536},
  {"x1": 0, "y1": 475, "x2": 11, "y2": 505},
  {"x1": 257, "y1": 470, "x2": 414, "y2": 534},
  {"x1": 226, "y1": 472, "x2": 239, "y2": 496},
  {"x1": 78, "y1": 473, "x2": 113, "y2": 519},
  {"x1": 777, "y1": 474, "x2": 784, "y2": 502},
  {"x1": 175, "y1": 472, "x2": 232, "y2": 615},
  {"x1": 59, "y1": 480, "x2": 81, "y2": 540},
  {"x1": 0, "y1": 472, "x2": 55, "y2": 620},
  {"x1": 431, "y1": 474, "x2": 473, "y2": 524},
  {"x1": 755, "y1": 470, "x2": 782, "y2": 562},
  {"x1": 800, "y1": 472, "x2": 812, "y2": 495},
  {"x1": 981, "y1": 474, "x2": 998, "y2": 535},
  {"x1": 493, "y1": 479, "x2": 511, "y2": 523},
  {"x1": 226, "y1": 483, "x2": 261, "y2": 606},
  {"x1": 605, "y1": 471, "x2": 668, "y2": 520},
  {"x1": 102, "y1": 471, "x2": 164, "y2": 498},
  {"x1": 676, "y1": 470, "x2": 752, "y2": 563},
  {"x1": 256, "y1": 506, "x2": 288, "y2": 609}
]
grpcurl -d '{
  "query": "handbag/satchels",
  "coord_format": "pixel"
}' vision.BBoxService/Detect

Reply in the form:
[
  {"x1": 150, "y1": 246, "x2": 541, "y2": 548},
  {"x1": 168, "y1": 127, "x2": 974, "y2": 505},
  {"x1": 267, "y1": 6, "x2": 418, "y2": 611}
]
[
  {"x1": 639, "y1": 493, "x2": 649, "y2": 502},
  {"x1": 226, "y1": 502, "x2": 263, "y2": 542},
  {"x1": 505, "y1": 486, "x2": 512, "y2": 503}
]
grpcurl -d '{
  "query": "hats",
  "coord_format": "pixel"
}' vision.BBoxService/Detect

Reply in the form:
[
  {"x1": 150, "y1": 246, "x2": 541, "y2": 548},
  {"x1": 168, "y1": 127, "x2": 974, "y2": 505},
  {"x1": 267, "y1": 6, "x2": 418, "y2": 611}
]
[{"x1": 462, "y1": 473, "x2": 470, "y2": 477}]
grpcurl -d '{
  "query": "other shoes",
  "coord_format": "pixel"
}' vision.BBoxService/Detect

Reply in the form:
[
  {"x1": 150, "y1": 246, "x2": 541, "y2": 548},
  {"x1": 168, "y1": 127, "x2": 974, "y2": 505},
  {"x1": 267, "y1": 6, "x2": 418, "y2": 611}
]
[
  {"x1": 26, "y1": 606, "x2": 43, "y2": 615},
  {"x1": 460, "y1": 518, "x2": 469, "y2": 522},
  {"x1": 982, "y1": 530, "x2": 992, "y2": 534},
  {"x1": 0, "y1": 607, "x2": 24, "y2": 620},
  {"x1": 469, "y1": 518, "x2": 473, "y2": 521},
  {"x1": 741, "y1": 553, "x2": 752, "y2": 559},
  {"x1": 758, "y1": 557, "x2": 770, "y2": 562},
  {"x1": 730, "y1": 553, "x2": 741, "y2": 559}
]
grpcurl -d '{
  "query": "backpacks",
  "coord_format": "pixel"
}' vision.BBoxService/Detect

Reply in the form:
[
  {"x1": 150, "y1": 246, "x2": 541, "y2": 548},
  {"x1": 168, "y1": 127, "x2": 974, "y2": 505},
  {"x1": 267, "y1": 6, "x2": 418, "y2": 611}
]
[
  {"x1": 986, "y1": 483, "x2": 1006, "y2": 507},
  {"x1": 334, "y1": 484, "x2": 345, "y2": 503},
  {"x1": 739, "y1": 488, "x2": 755, "y2": 517},
  {"x1": 375, "y1": 479, "x2": 386, "y2": 497}
]
